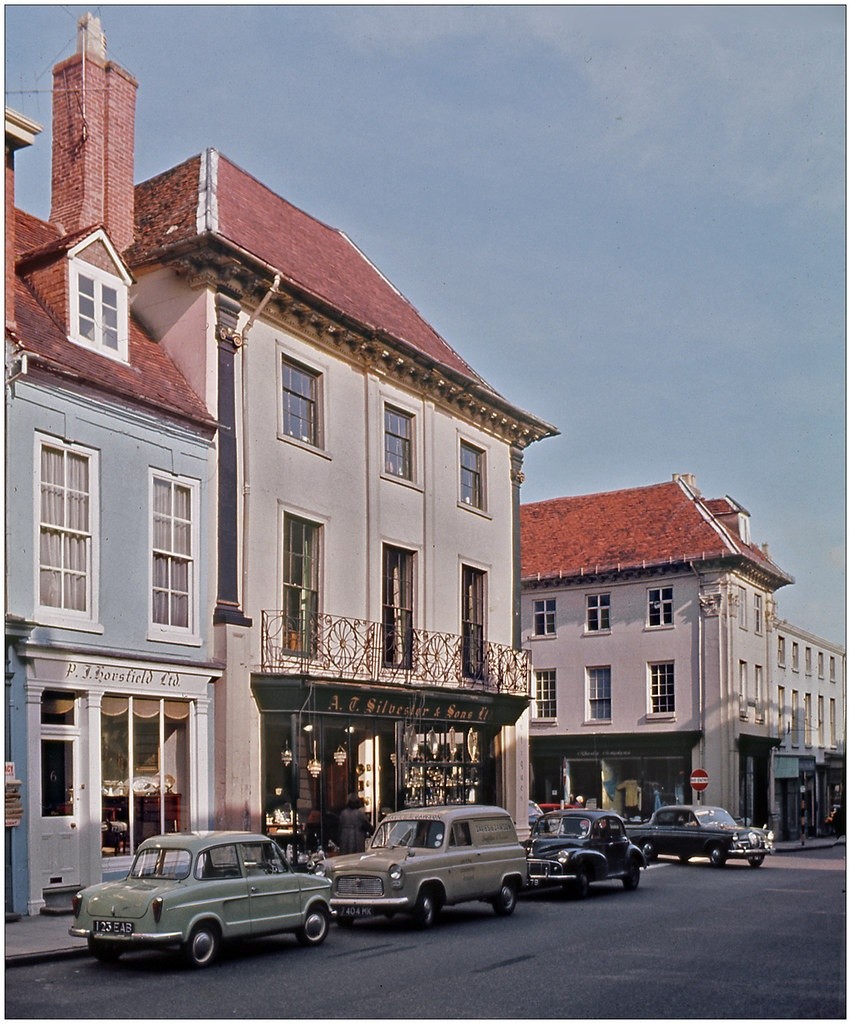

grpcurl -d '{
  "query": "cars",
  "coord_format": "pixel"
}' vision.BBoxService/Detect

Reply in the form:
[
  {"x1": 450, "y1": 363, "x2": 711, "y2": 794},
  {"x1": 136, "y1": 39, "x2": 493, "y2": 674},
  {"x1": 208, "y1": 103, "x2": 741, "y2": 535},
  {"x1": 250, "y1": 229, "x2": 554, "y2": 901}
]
[
  {"x1": 626, "y1": 805, "x2": 776, "y2": 867},
  {"x1": 516, "y1": 809, "x2": 655, "y2": 896},
  {"x1": 70, "y1": 830, "x2": 336, "y2": 971},
  {"x1": 528, "y1": 801, "x2": 588, "y2": 834}
]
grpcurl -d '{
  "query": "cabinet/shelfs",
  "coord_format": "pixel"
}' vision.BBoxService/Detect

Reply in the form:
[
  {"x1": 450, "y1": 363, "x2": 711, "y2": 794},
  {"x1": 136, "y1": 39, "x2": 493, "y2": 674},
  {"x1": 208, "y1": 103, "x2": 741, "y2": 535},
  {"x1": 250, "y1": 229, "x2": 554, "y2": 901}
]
[
  {"x1": 102, "y1": 794, "x2": 182, "y2": 846},
  {"x1": 402, "y1": 760, "x2": 481, "y2": 805}
]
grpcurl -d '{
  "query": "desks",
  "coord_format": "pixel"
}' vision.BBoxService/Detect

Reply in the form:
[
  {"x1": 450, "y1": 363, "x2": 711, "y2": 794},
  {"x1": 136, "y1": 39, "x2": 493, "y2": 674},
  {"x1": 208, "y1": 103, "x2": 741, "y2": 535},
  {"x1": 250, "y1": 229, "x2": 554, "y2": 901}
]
[{"x1": 268, "y1": 823, "x2": 317, "y2": 855}]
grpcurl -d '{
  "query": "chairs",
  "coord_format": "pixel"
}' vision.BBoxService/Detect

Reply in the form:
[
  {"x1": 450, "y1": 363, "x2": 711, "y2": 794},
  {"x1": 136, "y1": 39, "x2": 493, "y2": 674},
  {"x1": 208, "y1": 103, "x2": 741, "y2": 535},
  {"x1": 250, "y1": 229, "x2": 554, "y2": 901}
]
[{"x1": 103, "y1": 807, "x2": 128, "y2": 855}]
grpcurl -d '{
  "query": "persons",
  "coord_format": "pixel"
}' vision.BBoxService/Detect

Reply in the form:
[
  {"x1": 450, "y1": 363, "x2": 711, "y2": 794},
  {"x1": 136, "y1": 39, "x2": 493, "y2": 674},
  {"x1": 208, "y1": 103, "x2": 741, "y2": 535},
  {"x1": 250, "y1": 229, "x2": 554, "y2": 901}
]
[
  {"x1": 575, "y1": 796, "x2": 583, "y2": 806},
  {"x1": 338, "y1": 793, "x2": 373, "y2": 855}
]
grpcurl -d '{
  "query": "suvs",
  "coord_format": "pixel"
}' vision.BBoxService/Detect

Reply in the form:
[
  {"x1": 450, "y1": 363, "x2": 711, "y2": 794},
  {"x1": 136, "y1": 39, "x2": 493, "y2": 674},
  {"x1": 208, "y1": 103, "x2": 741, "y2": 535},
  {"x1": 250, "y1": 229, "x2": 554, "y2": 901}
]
[{"x1": 314, "y1": 806, "x2": 527, "y2": 932}]
